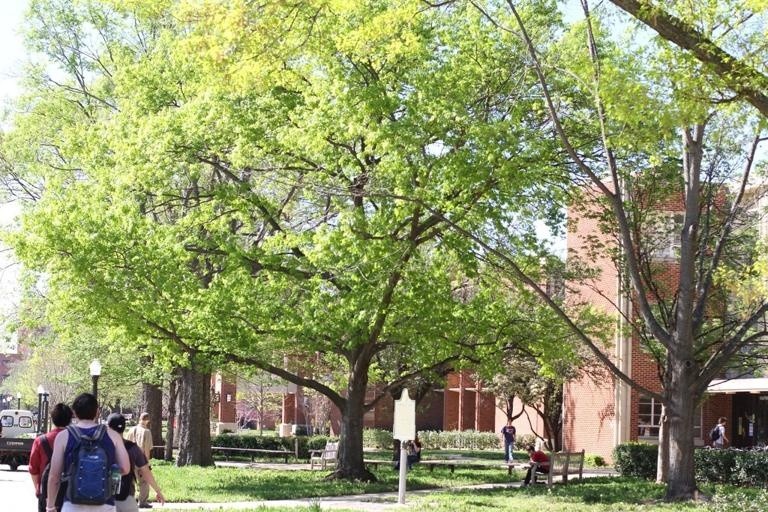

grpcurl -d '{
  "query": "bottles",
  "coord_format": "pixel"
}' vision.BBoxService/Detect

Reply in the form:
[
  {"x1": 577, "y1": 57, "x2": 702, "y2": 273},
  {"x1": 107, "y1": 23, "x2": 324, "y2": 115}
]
[{"x1": 109, "y1": 463, "x2": 121, "y2": 496}]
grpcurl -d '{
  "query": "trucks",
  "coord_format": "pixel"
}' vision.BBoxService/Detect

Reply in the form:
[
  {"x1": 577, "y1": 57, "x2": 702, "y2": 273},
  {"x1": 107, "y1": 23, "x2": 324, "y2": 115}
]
[{"x1": 0, "y1": 409, "x2": 43, "y2": 470}]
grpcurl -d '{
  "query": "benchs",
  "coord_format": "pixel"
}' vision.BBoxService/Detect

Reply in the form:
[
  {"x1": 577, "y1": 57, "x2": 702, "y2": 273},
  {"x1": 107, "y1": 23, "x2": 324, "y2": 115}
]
[
  {"x1": 531, "y1": 448, "x2": 585, "y2": 487},
  {"x1": 211, "y1": 445, "x2": 295, "y2": 464},
  {"x1": 363, "y1": 458, "x2": 457, "y2": 473}
]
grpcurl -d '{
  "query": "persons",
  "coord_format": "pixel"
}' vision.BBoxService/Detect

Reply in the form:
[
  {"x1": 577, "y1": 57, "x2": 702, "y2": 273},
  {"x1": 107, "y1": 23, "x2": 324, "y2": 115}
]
[
  {"x1": 28, "y1": 391, "x2": 164, "y2": 512},
  {"x1": 395, "y1": 436, "x2": 421, "y2": 470},
  {"x1": 713, "y1": 417, "x2": 728, "y2": 448},
  {"x1": 502, "y1": 419, "x2": 516, "y2": 464},
  {"x1": 520, "y1": 444, "x2": 549, "y2": 486}
]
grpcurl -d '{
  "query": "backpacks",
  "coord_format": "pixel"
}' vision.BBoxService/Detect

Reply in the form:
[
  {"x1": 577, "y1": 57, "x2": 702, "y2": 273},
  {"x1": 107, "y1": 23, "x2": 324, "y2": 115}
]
[
  {"x1": 65, "y1": 424, "x2": 112, "y2": 506},
  {"x1": 710, "y1": 424, "x2": 723, "y2": 441},
  {"x1": 111, "y1": 440, "x2": 136, "y2": 501},
  {"x1": 38, "y1": 434, "x2": 67, "y2": 511}
]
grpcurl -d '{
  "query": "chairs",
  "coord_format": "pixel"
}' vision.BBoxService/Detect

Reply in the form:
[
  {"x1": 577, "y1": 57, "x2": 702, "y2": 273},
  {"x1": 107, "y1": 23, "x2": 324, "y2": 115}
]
[{"x1": 307, "y1": 440, "x2": 341, "y2": 470}]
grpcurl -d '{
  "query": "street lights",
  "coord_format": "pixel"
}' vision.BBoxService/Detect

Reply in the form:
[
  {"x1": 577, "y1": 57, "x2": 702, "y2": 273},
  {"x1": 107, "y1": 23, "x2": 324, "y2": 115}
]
[
  {"x1": 89, "y1": 358, "x2": 101, "y2": 423},
  {"x1": 37, "y1": 384, "x2": 45, "y2": 431},
  {"x1": 3, "y1": 392, "x2": 22, "y2": 409}
]
